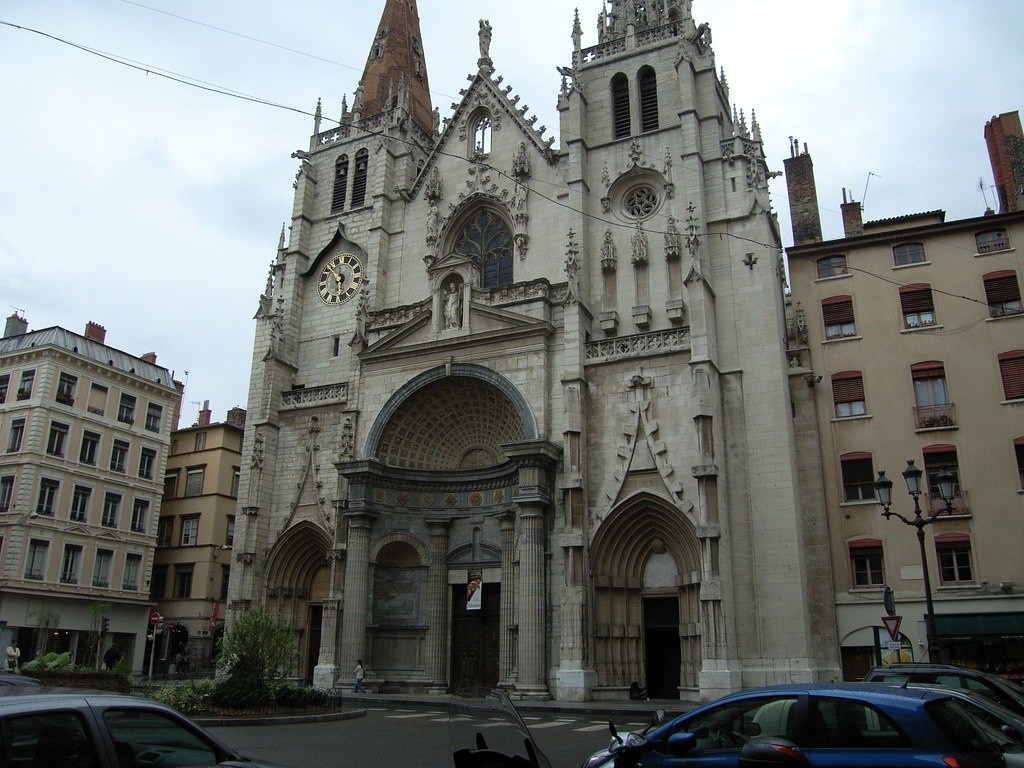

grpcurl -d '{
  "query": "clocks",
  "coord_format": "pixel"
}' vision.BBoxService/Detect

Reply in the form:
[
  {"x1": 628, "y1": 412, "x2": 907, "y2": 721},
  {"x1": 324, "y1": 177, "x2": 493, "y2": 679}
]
[{"x1": 317, "y1": 252, "x2": 363, "y2": 307}]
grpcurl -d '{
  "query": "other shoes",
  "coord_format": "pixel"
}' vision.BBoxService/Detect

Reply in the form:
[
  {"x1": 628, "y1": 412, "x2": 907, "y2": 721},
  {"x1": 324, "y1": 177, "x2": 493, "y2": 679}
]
[{"x1": 365, "y1": 691, "x2": 367, "y2": 694}]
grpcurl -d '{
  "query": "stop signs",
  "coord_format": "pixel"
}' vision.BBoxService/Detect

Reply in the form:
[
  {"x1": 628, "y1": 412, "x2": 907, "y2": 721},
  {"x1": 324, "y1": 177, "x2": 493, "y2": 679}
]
[
  {"x1": 168, "y1": 623, "x2": 173, "y2": 630},
  {"x1": 151, "y1": 613, "x2": 160, "y2": 625}
]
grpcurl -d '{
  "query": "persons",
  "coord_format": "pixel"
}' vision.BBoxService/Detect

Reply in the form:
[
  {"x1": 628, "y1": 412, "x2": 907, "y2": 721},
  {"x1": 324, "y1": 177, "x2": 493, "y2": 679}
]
[
  {"x1": 354, "y1": 660, "x2": 369, "y2": 695},
  {"x1": 103, "y1": 643, "x2": 121, "y2": 673},
  {"x1": 2, "y1": 639, "x2": 21, "y2": 676}
]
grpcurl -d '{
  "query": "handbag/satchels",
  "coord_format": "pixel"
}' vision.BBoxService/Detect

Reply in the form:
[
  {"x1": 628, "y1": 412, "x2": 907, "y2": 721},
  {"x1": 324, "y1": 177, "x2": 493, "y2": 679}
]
[
  {"x1": 363, "y1": 670, "x2": 365, "y2": 678},
  {"x1": 8, "y1": 660, "x2": 17, "y2": 668}
]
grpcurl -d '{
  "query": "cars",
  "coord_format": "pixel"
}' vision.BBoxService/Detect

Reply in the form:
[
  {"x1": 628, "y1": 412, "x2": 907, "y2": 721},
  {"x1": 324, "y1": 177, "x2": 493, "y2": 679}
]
[
  {"x1": 1, "y1": 673, "x2": 287, "y2": 768},
  {"x1": 583, "y1": 680, "x2": 1024, "y2": 768}
]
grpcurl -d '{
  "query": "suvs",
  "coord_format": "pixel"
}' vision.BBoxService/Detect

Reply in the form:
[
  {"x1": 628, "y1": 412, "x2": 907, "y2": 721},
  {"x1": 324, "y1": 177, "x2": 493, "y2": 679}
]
[{"x1": 861, "y1": 664, "x2": 1024, "y2": 715}]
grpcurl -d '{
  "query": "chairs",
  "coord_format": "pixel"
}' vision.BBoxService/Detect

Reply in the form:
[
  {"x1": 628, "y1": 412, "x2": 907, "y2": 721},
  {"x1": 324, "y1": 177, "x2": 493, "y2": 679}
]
[
  {"x1": 768, "y1": 701, "x2": 827, "y2": 744},
  {"x1": 31, "y1": 725, "x2": 86, "y2": 768}
]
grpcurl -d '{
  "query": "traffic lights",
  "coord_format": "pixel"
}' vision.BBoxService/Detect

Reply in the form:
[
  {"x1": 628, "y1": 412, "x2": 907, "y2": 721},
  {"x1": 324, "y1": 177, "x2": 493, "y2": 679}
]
[
  {"x1": 105, "y1": 618, "x2": 109, "y2": 631},
  {"x1": 155, "y1": 616, "x2": 164, "y2": 634}
]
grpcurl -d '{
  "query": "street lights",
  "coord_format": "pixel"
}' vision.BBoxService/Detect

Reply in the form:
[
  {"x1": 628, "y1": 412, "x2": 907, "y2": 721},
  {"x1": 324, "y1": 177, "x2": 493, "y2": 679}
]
[{"x1": 873, "y1": 458, "x2": 956, "y2": 666}]
